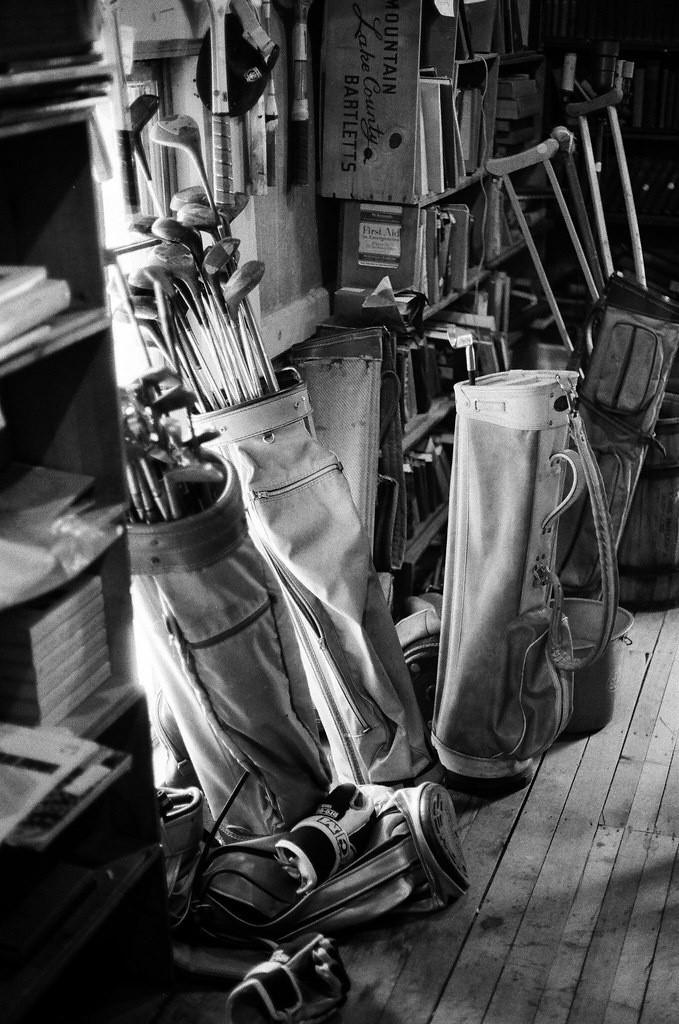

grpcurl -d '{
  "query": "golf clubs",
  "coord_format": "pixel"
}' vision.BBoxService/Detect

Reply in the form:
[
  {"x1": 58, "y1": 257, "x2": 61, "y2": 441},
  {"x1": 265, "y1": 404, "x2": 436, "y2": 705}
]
[
  {"x1": 104, "y1": 94, "x2": 281, "y2": 521},
  {"x1": 446, "y1": 325, "x2": 476, "y2": 385}
]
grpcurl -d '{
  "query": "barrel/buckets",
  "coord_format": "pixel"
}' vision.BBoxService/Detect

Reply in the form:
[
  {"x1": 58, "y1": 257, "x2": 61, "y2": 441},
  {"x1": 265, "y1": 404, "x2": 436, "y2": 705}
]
[
  {"x1": 549, "y1": 37, "x2": 620, "y2": 118},
  {"x1": 549, "y1": 596, "x2": 635, "y2": 734}
]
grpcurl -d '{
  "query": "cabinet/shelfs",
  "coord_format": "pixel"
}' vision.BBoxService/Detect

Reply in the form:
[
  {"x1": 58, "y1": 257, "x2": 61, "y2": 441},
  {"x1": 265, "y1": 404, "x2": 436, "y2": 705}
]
[
  {"x1": 318, "y1": 1, "x2": 547, "y2": 623},
  {"x1": 0, "y1": 99, "x2": 170, "y2": 1024}
]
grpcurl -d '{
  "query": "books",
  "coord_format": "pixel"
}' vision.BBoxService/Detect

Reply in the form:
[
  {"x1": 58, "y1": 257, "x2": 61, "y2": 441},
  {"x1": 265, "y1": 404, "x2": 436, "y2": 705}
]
[
  {"x1": 0, "y1": 573, "x2": 132, "y2": 850},
  {"x1": 0, "y1": 263, "x2": 73, "y2": 363},
  {"x1": 396, "y1": 0, "x2": 679, "y2": 542}
]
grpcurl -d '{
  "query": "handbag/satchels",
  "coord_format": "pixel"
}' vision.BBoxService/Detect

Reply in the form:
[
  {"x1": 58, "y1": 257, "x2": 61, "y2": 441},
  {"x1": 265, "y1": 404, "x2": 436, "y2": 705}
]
[{"x1": 202, "y1": 782, "x2": 471, "y2": 938}]
